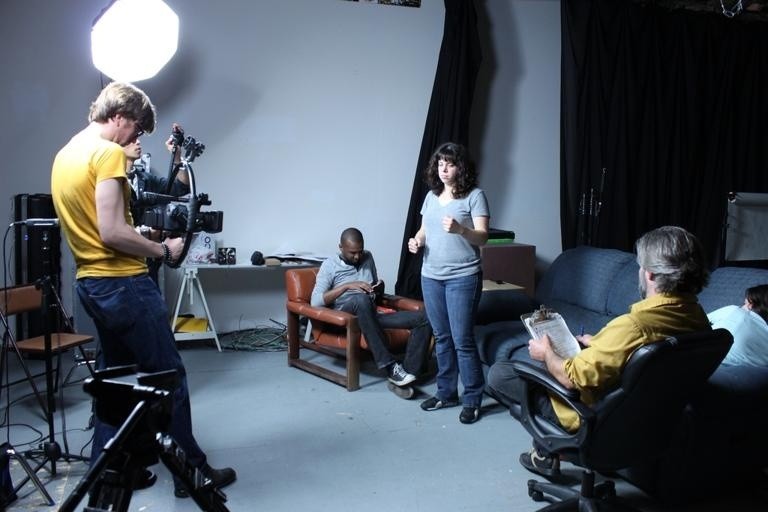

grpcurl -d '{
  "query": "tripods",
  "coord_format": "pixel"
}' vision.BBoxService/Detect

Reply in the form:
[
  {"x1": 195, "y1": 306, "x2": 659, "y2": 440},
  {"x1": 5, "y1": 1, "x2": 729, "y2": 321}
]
[{"x1": 0, "y1": 233, "x2": 90, "y2": 507}]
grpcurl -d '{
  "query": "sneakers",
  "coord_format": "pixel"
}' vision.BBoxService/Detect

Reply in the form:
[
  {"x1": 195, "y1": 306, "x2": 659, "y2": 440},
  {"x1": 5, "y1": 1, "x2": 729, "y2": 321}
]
[
  {"x1": 420, "y1": 393, "x2": 461, "y2": 412},
  {"x1": 458, "y1": 406, "x2": 483, "y2": 425},
  {"x1": 388, "y1": 379, "x2": 416, "y2": 401},
  {"x1": 386, "y1": 364, "x2": 418, "y2": 388},
  {"x1": 517, "y1": 447, "x2": 563, "y2": 480},
  {"x1": 173, "y1": 460, "x2": 238, "y2": 499}
]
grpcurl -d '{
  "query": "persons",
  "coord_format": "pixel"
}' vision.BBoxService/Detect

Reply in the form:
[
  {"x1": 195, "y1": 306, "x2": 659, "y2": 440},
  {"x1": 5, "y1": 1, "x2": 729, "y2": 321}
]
[
  {"x1": 406, "y1": 141, "x2": 490, "y2": 425},
  {"x1": 311, "y1": 226, "x2": 432, "y2": 400},
  {"x1": 486, "y1": 223, "x2": 714, "y2": 485},
  {"x1": 119, "y1": 135, "x2": 192, "y2": 295},
  {"x1": 51, "y1": 80, "x2": 236, "y2": 499},
  {"x1": 704, "y1": 281, "x2": 766, "y2": 372}
]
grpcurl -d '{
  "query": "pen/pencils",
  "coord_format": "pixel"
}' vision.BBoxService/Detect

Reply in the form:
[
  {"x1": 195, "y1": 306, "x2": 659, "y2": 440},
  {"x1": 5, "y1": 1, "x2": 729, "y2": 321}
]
[{"x1": 581, "y1": 326, "x2": 585, "y2": 349}]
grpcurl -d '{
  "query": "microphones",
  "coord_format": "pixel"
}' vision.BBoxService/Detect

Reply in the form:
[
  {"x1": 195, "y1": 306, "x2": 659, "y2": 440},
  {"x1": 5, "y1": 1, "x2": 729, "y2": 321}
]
[{"x1": 24, "y1": 217, "x2": 59, "y2": 227}]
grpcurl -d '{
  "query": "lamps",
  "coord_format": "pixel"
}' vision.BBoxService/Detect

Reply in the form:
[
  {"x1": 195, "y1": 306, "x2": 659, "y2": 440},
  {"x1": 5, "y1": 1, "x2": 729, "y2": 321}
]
[{"x1": 90, "y1": 0, "x2": 179, "y2": 85}]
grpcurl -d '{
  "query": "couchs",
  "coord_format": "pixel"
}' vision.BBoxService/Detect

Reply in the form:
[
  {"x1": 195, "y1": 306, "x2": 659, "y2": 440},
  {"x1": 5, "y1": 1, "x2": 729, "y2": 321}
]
[{"x1": 472, "y1": 245, "x2": 768, "y2": 408}]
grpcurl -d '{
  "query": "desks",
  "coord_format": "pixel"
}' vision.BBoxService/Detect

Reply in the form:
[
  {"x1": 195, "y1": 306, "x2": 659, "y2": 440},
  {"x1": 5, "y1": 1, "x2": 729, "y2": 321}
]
[
  {"x1": 171, "y1": 260, "x2": 324, "y2": 353},
  {"x1": 481, "y1": 279, "x2": 527, "y2": 292},
  {"x1": 472, "y1": 243, "x2": 536, "y2": 302}
]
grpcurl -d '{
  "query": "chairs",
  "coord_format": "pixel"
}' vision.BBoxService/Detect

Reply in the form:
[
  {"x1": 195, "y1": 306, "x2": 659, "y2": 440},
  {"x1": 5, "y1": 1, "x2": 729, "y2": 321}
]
[
  {"x1": 0, "y1": 277, "x2": 96, "y2": 411},
  {"x1": 284, "y1": 267, "x2": 434, "y2": 392},
  {"x1": 510, "y1": 329, "x2": 734, "y2": 512}
]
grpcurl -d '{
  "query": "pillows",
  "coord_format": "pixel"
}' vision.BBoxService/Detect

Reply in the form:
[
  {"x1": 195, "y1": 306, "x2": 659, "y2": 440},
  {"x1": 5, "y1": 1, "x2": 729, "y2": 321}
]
[
  {"x1": 696, "y1": 266, "x2": 768, "y2": 315},
  {"x1": 607, "y1": 252, "x2": 644, "y2": 315},
  {"x1": 536, "y1": 246, "x2": 637, "y2": 315}
]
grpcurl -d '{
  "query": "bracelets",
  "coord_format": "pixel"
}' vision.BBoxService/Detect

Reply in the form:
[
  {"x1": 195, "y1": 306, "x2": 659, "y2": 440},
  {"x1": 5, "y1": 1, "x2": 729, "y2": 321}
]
[
  {"x1": 460, "y1": 226, "x2": 467, "y2": 235},
  {"x1": 160, "y1": 241, "x2": 173, "y2": 265},
  {"x1": 172, "y1": 160, "x2": 187, "y2": 172}
]
